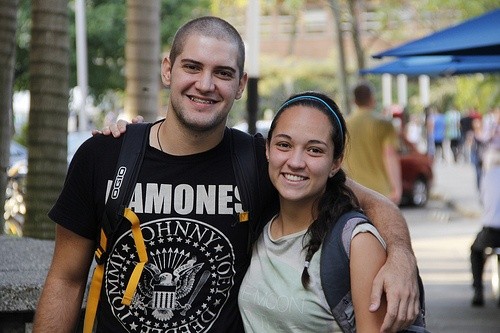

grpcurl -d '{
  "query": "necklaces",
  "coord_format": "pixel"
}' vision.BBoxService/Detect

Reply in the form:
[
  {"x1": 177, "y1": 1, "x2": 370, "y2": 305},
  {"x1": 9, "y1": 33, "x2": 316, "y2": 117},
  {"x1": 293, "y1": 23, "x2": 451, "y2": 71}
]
[{"x1": 157, "y1": 120, "x2": 164, "y2": 152}]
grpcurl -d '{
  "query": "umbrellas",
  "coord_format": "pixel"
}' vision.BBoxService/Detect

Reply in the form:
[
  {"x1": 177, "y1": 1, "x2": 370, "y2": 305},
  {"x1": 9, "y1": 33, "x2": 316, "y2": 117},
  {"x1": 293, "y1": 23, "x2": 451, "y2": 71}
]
[
  {"x1": 371, "y1": 8, "x2": 500, "y2": 59},
  {"x1": 360, "y1": 56, "x2": 500, "y2": 106}
]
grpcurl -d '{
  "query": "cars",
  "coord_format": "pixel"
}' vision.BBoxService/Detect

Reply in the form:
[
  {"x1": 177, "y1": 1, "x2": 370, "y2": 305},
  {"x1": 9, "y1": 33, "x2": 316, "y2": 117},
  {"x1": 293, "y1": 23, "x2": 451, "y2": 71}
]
[{"x1": 384, "y1": 104, "x2": 434, "y2": 208}]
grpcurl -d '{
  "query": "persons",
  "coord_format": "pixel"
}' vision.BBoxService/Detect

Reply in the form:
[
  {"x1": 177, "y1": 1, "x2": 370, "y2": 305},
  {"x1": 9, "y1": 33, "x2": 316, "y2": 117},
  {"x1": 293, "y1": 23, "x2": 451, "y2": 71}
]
[
  {"x1": 404, "y1": 94, "x2": 500, "y2": 307},
  {"x1": 92, "y1": 92, "x2": 387, "y2": 333},
  {"x1": 340, "y1": 84, "x2": 403, "y2": 205},
  {"x1": 32, "y1": 17, "x2": 420, "y2": 333}
]
